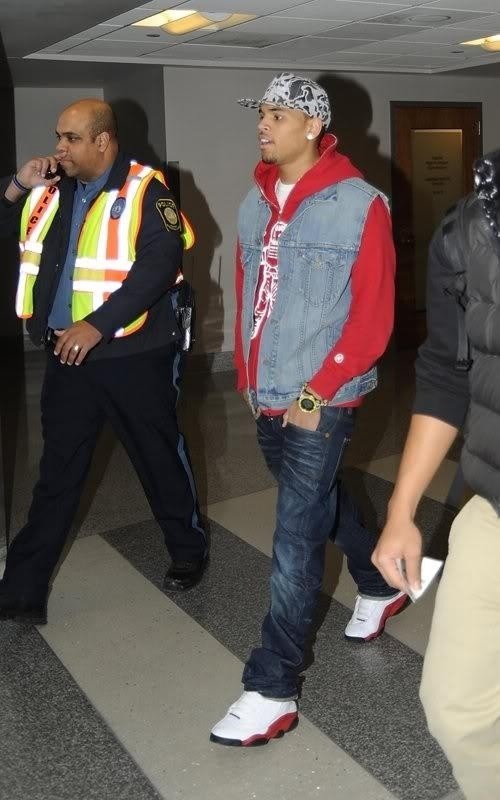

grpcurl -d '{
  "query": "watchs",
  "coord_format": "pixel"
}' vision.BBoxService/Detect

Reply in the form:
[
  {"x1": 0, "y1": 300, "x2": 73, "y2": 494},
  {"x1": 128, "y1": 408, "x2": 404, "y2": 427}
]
[{"x1": 298, "y1": 386, "x2": 329, "y2": 414}]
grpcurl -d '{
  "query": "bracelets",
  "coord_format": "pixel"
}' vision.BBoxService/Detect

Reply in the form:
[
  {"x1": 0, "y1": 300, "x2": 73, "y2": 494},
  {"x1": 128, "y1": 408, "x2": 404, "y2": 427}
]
[{"x1": 12, "y1": 173, "x2": 29, "y2": 192}]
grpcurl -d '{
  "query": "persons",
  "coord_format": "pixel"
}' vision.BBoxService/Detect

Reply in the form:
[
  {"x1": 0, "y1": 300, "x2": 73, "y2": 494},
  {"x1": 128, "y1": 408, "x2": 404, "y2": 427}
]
[
  {"x1": 209, "y1": 68, "x2": 413, "y2": 748},
  {"x1": 0, "y1": 98, "x2": 207, "y2": 619},
  {"x1": 369, "y1": 148, "x2": 498, "y2": 800}
]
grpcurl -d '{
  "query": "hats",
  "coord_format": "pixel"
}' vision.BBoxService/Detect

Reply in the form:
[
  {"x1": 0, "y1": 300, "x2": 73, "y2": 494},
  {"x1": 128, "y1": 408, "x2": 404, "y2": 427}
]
[{"x1": 235, "y1": 71, "x2": 331, "y2": 133}]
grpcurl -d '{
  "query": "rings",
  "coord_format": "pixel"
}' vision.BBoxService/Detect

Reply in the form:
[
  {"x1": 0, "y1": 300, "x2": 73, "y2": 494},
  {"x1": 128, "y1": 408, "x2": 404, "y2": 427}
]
[{"x1": 72, "y1": 344, "x2": 79, "y2": 351}]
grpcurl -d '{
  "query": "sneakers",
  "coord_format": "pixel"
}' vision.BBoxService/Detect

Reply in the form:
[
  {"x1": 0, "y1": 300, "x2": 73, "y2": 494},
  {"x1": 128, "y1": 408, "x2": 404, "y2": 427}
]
[
  {"x1": 1, "y1": 580, "x2": 51, "y2": 627},
  {"x1": 209, "y1": 689, "x2": 301, "y2": 747},
  {"x1": 163, "y1": 549, "x2": 209, "y2": 592},
  {"x1": 343, "y1": 589, "x2": 414, "y2": 642}
]
